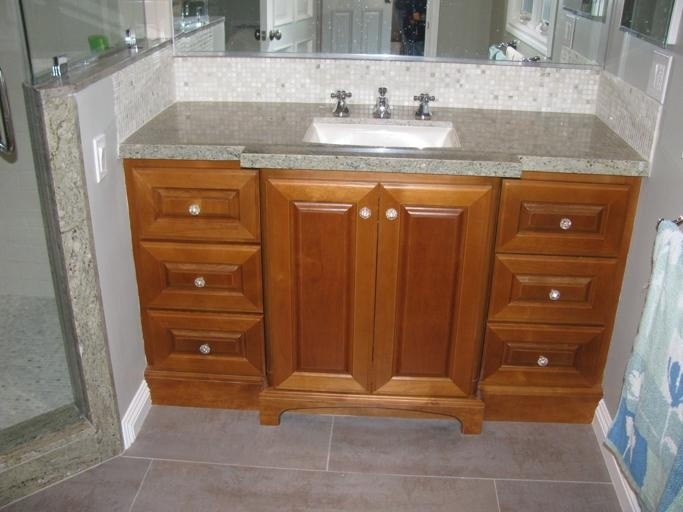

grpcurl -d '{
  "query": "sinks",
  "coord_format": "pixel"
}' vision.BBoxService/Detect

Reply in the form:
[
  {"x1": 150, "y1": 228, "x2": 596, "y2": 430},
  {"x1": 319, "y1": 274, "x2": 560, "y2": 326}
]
[{"x1": 302, "y1": 117, "x2": 461, "y2": 150}]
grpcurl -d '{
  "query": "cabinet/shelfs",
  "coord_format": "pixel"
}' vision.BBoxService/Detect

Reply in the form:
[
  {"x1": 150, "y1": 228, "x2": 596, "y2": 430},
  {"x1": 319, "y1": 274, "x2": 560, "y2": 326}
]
[{"x1": 124, "y1": 158, "x2": 641, "y2": 435}]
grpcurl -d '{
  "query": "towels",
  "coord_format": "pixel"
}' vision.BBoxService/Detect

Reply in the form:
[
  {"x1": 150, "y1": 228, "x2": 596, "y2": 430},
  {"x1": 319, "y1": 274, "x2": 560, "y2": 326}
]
[
  {"x1": 489, "y1": 45, "x2": 508, "y2": 60},
  {"x1": 506, "y1": 46, "x2": 523, "y2": 61},
  {"x1": 603, "y1": 220, "x2": 683, "y2": 512}
]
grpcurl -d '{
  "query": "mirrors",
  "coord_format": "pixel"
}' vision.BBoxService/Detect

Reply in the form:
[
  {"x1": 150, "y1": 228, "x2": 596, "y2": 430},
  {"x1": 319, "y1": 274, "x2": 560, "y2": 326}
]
[{"x1": 171, "y1": 0, "x2": 614, "y2": 70}]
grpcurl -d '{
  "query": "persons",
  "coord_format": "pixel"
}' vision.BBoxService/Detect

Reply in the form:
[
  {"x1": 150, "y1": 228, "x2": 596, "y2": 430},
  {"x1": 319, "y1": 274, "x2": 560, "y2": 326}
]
[{"x1": 393, "y1": 1, "x2": 427, "y2": 58}]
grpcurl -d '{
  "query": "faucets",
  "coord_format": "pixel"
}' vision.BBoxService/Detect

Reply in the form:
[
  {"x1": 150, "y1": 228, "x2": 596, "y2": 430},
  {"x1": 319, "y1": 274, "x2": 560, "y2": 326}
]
[{"x1": 372, "y1": 88, "x2": 391, "y2": 119}]
